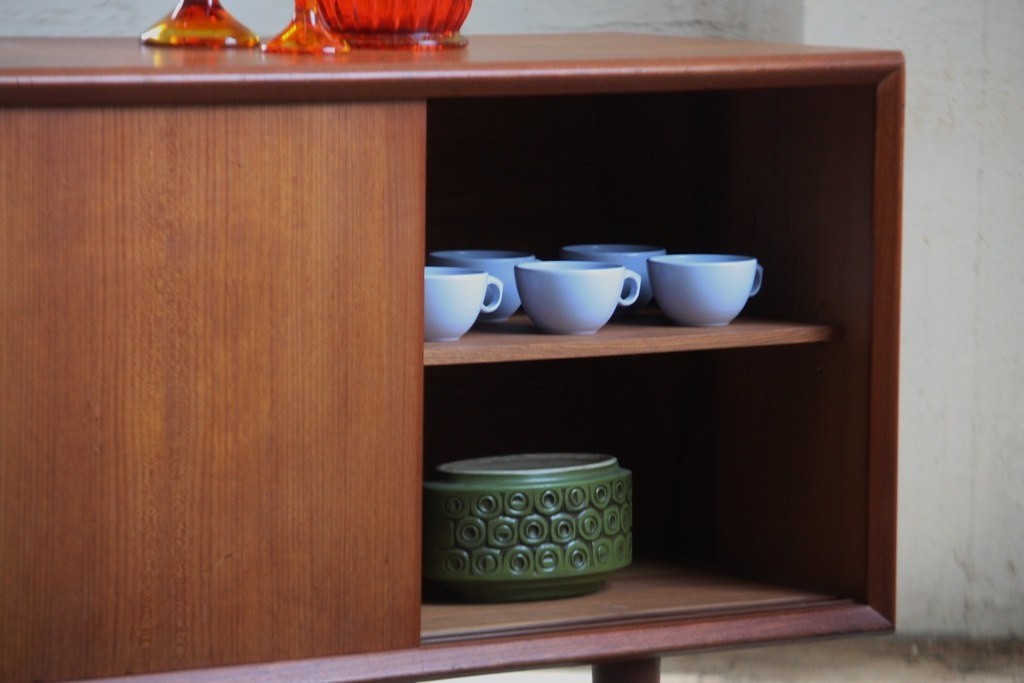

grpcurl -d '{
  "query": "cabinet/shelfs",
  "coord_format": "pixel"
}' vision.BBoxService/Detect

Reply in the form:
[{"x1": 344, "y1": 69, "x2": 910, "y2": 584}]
[{"x1": 0, "y1": 32, "x2": 908, "y2": 683}]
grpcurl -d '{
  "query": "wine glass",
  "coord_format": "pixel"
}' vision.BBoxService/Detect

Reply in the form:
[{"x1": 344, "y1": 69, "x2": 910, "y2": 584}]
[
  {"x1": 139, "y1": 0, "x2": 261, "y2": 50},
  {"x1": 264, "y1": 0, "x2": 351, "y2": 54}
]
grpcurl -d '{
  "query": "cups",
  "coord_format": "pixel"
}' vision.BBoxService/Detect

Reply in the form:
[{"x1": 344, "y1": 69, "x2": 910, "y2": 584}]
[
  {"x1": 430, "y1": 251, "x2": 541, "y2": 321},
  {"x1": 515, "y1": 260, "x2": 642, "y2": 335},
  {"x1": 424, "y1": 267, "x2": 503, "y2": 342},
  {"x1": 315, "y1": 0, "x2": 474, "y2": 52},
  {"x1": 561, "y1": 245, "x2": 668, "y2": 315},
  {"x1": 647, "y1": 254, "x2": 763, "y2": 327}
]
